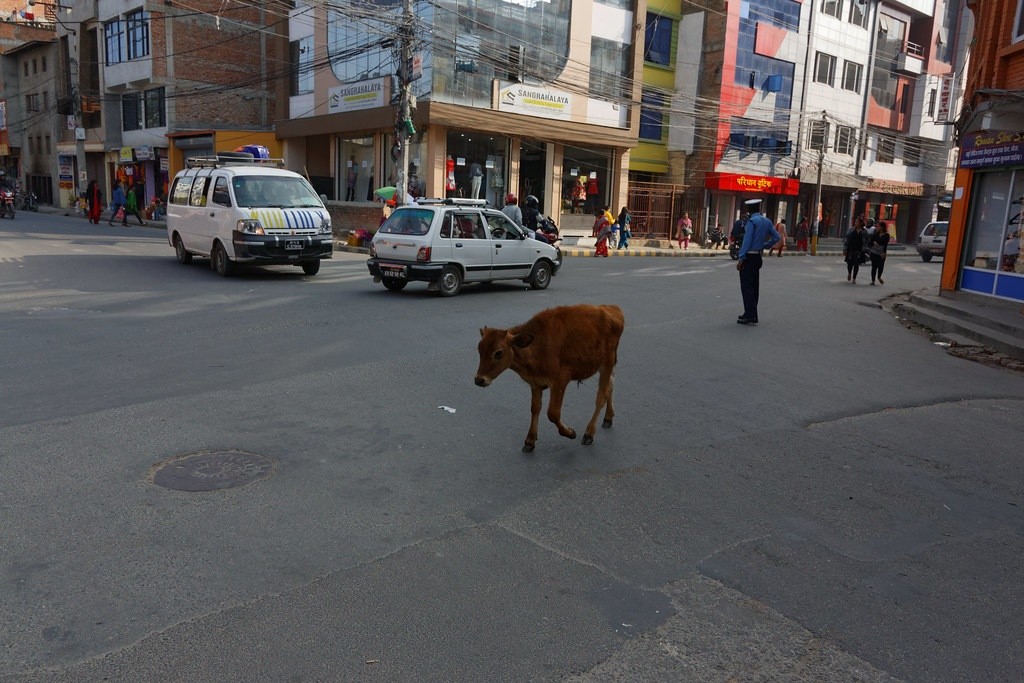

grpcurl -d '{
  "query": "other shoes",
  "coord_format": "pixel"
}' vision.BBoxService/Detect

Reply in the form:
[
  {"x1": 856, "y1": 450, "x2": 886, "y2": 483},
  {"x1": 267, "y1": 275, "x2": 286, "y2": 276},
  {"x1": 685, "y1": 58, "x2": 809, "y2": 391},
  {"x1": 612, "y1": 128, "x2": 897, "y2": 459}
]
[
  {"x1": 870, "y1": 282, "x2": 874, "y2": 285},
  {"x1": 877, "y1": 278, "x2": 883, "y2": 284},
  {"x1": 847, "y1": 274, "x2": 851, "y2": 281},
  {"x1": 852, "y1": 280, "x2": 856, "y2": 284}
]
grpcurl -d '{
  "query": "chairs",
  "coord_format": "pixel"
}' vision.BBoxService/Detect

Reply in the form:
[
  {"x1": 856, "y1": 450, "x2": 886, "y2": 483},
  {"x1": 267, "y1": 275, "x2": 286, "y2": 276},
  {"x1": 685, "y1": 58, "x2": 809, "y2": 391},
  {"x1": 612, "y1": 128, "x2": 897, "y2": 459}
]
[{"x1": 442, "y1": 216, "x2": 478, "y2": 238}]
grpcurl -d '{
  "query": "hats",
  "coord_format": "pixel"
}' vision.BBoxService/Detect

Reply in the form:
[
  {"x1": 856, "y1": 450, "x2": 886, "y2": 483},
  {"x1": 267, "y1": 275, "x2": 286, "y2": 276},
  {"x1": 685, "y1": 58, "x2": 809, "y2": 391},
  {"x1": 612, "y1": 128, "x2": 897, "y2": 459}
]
[{"x1": 744, "y1": 199, "x2": 762, "y2": 207}]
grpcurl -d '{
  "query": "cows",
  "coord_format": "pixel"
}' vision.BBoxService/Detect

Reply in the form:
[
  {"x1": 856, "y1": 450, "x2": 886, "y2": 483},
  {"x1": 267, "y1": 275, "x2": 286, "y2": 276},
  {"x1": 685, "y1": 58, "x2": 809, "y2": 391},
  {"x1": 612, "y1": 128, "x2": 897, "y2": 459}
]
[{"x1": 475, "y1": 304, "x2": 624, "y2": 453}]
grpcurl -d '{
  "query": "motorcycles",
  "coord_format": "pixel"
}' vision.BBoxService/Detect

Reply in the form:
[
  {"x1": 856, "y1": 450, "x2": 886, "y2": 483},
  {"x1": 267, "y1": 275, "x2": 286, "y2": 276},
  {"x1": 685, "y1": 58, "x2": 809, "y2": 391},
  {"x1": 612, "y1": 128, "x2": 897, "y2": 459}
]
[
  {"x1": 0, "y1": 169, "x2": 39, "y2": 220},
  {"x1": 499, "y1": 216, "x2": 561, "y2": 274}
]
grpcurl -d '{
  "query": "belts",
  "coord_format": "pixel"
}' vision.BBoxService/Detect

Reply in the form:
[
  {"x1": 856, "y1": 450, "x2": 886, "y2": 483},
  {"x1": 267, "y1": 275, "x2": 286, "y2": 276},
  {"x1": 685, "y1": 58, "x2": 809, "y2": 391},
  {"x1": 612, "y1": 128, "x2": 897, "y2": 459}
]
[{"x1": 747, "y1": 251, "x2": 762, "y2": 254}]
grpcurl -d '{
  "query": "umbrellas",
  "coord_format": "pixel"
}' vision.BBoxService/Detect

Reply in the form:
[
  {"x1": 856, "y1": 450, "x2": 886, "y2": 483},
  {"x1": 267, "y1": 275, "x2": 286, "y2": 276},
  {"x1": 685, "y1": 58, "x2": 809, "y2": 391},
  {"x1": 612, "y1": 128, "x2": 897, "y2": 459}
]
[{"x1": 374, "y1": 186, "x2": 414, "y2": 206}]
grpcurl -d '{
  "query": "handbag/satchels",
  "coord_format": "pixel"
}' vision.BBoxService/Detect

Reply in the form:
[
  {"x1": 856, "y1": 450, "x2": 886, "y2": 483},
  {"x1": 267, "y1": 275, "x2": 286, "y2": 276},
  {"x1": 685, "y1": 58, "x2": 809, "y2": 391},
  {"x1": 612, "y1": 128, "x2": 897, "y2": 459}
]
[
  {"x1": 623, "y1": 224, "x2": 630, "y2": 232},
  {"x1": 115, "y1": 208, "x2": 124, "y2": 219},
  {"x1": 682, "y1": 227, "x2": 693, "y2": 236}
]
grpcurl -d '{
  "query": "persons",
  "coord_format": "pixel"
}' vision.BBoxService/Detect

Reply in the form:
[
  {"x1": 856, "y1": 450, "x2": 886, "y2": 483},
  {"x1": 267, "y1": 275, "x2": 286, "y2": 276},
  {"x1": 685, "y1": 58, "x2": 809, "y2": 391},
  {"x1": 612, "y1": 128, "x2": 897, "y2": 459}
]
[
  {"x1": 501, "y1": 195, "x2": 554, "y2": 236},
  {"x1": 736, "y1": 199, "x2": 780, "y2": 324},
  {"x1": 446, "y1": 155, "x2": 456, "y2": 191},
  {"x1": 87, "y1": 180, "x2": 102, "y2": 224},
  {"x1": 476, "y1": 217, "x2": 507, "y2": 239},
  {"x1": 469, "y1": 160, "x2": 484, "y2": 199},
  {"x1": 379, "y1": 203, "x2": 394, "y2": 229},
  {"x1": 710, "y1": 216, "x2": 810, "y2": 256},
  {"x1": 570, "y1": 182, "x2": 581, "y2": 214},
  {"x1": 677, "y1": 211, "x2": 693, "y2": 250},
  {"x1": 109, "y1": 179, "x2": 166, "y2": 227},
  {"x1": 843, "y1": 211, "x2": 890, "y2": 286},
  {"x1": 593, "y1": 206, "x2": 632, "y2": 257},
  {"x1": 586, "y1": 172, "x2": 600, "y2": 195},
  {"x1": 345, "y1": 155, "x2": 359, "y2": 201},
  {"x1": 0, "y1": 171, "x2": 16, "y2": 196}
]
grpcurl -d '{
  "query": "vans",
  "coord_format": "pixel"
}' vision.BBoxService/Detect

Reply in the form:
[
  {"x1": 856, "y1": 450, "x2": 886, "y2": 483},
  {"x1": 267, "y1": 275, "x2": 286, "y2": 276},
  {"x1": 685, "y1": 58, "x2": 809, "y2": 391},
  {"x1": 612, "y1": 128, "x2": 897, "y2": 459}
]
[{"x1": 168, "y1": 152, "x2": 333, "y2": 277}]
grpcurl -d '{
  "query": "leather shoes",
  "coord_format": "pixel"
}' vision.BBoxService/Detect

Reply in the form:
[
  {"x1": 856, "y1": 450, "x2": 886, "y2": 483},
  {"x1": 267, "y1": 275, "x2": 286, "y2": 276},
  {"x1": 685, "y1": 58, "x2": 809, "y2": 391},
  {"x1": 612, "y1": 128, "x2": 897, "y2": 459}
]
[
  {"x1": 738, "y1": 314, "x2": 746, "y2": 319},
  {"x1": 737, "y1": 318, "x2": 758, "y2": 325}
]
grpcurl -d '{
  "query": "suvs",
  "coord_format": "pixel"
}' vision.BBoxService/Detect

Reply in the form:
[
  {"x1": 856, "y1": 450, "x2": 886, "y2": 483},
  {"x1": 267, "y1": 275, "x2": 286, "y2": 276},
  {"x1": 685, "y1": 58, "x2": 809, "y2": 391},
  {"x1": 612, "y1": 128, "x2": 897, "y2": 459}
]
[
  {"x1": 916, "y1": 221, "x2": 950, "y2": 261},
  {"x1": 366, "y1": 198, "x2": 561, "y2": 296}
]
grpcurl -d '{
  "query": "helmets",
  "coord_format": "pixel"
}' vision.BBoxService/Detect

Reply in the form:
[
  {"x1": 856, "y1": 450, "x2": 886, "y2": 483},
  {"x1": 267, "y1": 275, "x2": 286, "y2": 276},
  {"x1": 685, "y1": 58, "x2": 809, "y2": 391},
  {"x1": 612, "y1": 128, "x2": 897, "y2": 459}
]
[
  {"x1": 505, "y1": 194, "x2": 517, "y2": 205},
  {"x1": 524, "y1": 195, "x2": 540, "y2": 208}
]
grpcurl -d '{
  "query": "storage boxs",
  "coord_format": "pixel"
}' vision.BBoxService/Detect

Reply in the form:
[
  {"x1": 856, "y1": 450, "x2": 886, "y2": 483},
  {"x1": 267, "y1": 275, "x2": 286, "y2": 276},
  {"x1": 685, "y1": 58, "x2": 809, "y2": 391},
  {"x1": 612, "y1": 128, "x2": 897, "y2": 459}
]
[{"x1": 348, "y1": 234, "x2": 372, "y2": 248}]
